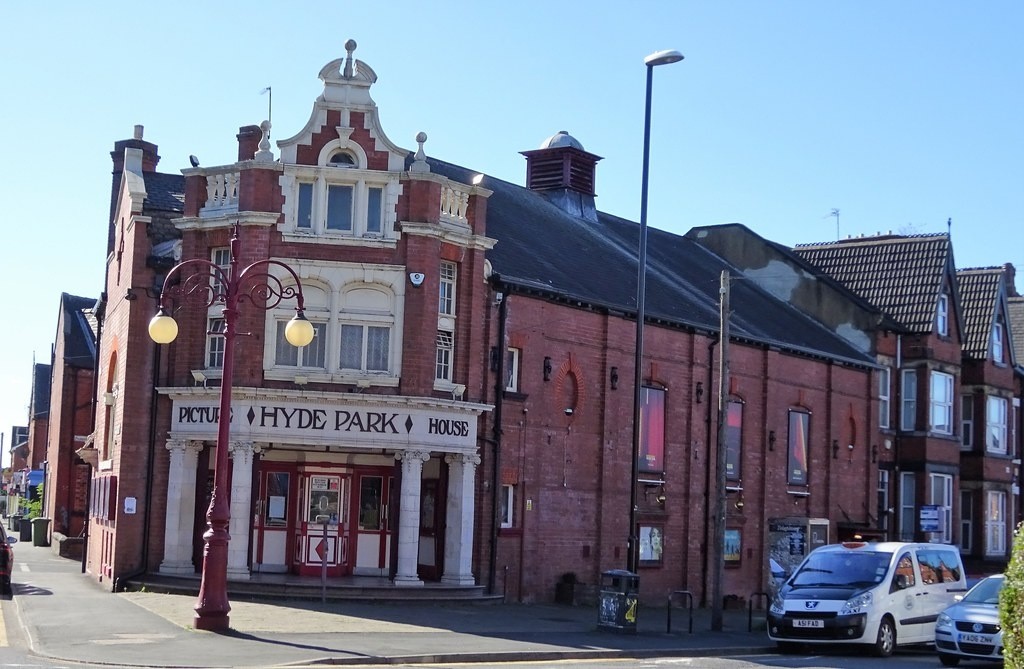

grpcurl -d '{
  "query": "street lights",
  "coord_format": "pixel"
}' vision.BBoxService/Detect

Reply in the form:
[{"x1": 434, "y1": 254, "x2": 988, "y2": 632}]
[
  {"x1": 625, "y1": 49, "x2": 685, "y2": 589},
  {"x1": 149, "y1": 219, "x2": 315, "y2": 631}
]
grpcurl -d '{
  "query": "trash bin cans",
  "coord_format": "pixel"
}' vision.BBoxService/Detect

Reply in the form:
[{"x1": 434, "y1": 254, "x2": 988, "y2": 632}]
[
  {"x1": 14, "y1": 515, "x2": 23, "y2": 531},
  {"x1": 598, "y1": 569, "x2": 641, "y2": 637},
  {"x1": 19, "y1": 519, "x2": 33, "y2": 542},
  {"x1": 30, "y1": 517, "x2": 52, "y2": 546}
]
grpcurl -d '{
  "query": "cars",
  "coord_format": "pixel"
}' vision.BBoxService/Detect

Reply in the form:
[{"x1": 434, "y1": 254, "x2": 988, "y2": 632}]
[
  {"x1": 0, "y1": 520, "x2": 17, "y2": 593},
  {"x1": 933, "y1": 573, "x2": 1008, "y2": 666}
]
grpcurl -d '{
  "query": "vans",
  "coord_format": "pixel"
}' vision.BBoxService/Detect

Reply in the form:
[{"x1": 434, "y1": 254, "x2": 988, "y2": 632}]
[{"x1": 766, "y1": 543, "x2": 966, "y2": 659}]
